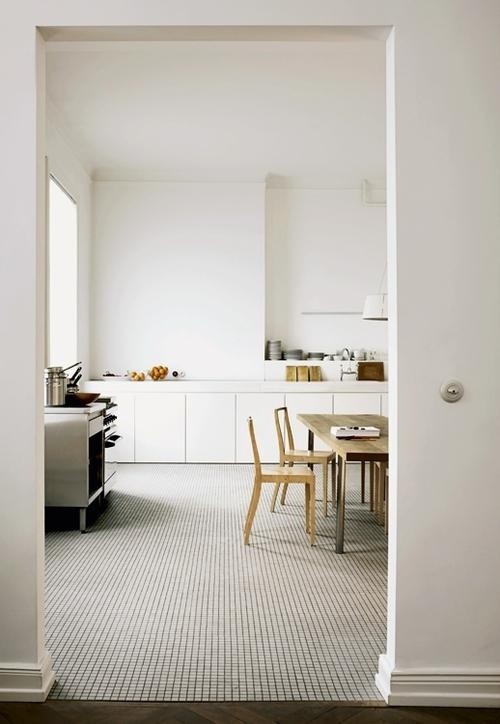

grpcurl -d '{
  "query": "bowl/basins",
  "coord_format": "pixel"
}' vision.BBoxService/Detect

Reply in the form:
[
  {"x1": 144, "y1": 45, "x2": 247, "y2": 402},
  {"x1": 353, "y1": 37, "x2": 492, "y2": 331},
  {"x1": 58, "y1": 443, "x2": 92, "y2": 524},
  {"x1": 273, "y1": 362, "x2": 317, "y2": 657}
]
[{"x1": 333, "y1": 355, "x2": 343, "y2": 361}]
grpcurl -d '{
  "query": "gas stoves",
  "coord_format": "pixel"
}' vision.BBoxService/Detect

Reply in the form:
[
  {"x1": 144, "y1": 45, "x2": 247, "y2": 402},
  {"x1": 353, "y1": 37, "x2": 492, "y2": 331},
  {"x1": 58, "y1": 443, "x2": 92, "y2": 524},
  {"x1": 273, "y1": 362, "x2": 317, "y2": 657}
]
[{"x1": 106, "y1": 403, "x2": 117, "y2": 410}]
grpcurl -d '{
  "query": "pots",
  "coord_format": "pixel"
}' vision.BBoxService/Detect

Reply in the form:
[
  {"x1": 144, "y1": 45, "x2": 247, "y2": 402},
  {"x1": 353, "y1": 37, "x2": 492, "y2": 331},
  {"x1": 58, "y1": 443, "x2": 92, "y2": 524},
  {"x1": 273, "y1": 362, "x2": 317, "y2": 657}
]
[
  {"x1": 67, "y1": 366, "x2": 83, "y2": 392},
  {"x1": 65, "y1": 392, "x2": 100, "y2": 406},
  {"x1": 44, "y1": 377, "x2": 67, "y2": 407},
  {"x1": 44, "y1": 361, "x2": 82, "y2": 378}
]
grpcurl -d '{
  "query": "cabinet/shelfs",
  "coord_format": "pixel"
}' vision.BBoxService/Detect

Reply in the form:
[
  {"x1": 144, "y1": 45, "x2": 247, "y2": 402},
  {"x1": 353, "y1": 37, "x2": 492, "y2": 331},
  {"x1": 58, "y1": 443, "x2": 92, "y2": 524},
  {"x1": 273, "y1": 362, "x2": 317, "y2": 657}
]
[
  {"x1": 333, "y1": 394, "x2": 381, "y2": 464},
  {"x1": 236, "y1": 394, "x2": 285, "y2": 464},
  {"x1": 184, "y1": 394, "x2": 236, "y2": 464},
  {"x1": 84, "y1": 393, "x2": 135, "y2": 464},
  {"x1": 381, "y1": 394, "x2": 389, "y2": 420},
  {"x1": 135, "y1": 393, "x2": 185, "y2": 463},
  {"x1": 285, "y1": 394, "x2": 333, "y2": 464}
]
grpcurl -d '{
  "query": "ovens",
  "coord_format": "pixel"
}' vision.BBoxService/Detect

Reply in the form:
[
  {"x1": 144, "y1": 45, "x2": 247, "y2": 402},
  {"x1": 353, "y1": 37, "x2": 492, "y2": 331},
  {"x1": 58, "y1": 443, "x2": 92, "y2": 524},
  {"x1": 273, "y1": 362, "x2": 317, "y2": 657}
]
[{"x1": 104, "y1": 424, "x2": 124, "y2": 498}]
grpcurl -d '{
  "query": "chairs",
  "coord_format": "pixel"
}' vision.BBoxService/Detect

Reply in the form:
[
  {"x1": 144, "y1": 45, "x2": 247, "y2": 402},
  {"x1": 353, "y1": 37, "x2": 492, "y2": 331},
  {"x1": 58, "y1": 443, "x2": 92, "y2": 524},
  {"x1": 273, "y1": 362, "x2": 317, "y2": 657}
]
[
  {"x1": 243, "y1": 415, "x2": 317, "y2": 547},
  {"x1": 270, "y1": 407, "x2": 337, "y2": 518}
]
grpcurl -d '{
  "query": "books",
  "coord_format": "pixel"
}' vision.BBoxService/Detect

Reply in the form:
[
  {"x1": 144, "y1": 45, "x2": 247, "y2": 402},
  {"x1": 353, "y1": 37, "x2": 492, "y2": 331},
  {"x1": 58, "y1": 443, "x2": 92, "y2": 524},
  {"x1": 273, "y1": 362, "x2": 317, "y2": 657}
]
[{"x1": 331, "y1": 426, "x2": 379, "y2": 437}]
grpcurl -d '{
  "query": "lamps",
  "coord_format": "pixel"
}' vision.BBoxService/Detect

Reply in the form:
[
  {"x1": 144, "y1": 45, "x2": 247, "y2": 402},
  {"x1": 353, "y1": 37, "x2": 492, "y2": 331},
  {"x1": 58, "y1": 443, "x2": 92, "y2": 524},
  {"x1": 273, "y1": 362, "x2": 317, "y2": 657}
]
[{"x1": 362, "y1": 294, "x2": 389, "y2": 321}]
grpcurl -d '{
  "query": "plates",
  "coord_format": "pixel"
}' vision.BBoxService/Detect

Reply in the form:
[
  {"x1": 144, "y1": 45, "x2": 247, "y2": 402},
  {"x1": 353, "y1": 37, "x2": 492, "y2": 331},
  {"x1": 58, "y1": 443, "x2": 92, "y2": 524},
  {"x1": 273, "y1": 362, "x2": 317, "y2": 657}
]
[
  {"x1": 267, "y1": 340, "x2": 282, "y2": 361},
  {"x1": 282, "y1": 349, "x2": 304, "y2": 360},
  {"x1": 101, "y1": 376, "x2": 129, "y2": 382},
  {"x1": 307, "y1": 352, "x2": 325, "y2": 361}
]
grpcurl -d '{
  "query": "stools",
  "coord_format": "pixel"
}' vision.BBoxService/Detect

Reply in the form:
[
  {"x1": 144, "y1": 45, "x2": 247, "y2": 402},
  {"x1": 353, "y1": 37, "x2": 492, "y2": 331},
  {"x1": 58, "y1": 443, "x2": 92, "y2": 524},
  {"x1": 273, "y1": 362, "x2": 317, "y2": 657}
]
[{"x1": 374, "y1": 461, "x2": 388, "y2": 526}]
